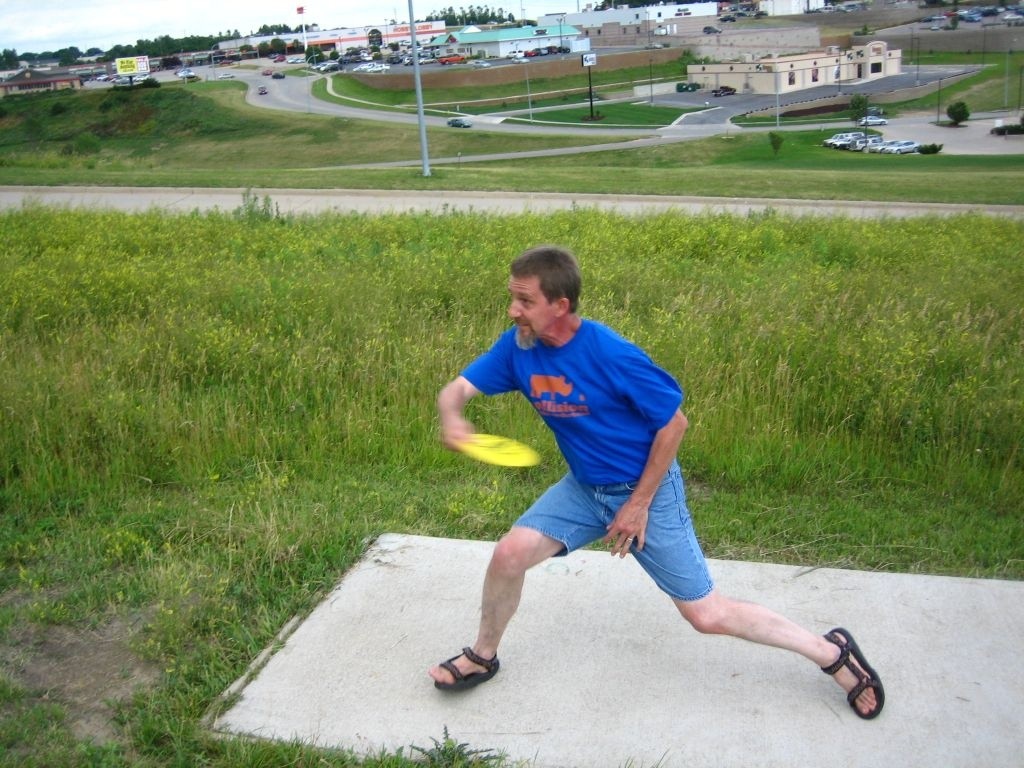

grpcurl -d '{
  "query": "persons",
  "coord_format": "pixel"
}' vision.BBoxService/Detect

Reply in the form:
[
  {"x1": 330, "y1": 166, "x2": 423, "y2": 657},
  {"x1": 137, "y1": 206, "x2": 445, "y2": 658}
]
[{"x1": 427, "y1": 248, "x2": 885, "y2": 721}]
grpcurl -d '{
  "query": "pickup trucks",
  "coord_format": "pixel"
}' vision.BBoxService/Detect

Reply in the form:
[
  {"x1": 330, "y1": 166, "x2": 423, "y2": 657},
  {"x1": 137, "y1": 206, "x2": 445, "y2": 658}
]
[{"x1": 712, "y1": 86, "x2": 736, "y2": 97}]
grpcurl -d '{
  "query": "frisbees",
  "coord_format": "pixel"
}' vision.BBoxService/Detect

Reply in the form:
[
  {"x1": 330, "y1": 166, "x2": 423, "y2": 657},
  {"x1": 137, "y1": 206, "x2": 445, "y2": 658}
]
[{"x1": 455, "y1": 432, "x2": 543, "y2": 469}]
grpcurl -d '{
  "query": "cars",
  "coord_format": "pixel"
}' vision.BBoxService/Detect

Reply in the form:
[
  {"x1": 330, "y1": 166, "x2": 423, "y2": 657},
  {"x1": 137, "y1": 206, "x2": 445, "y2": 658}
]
[
  {"x1": 269, "y1": 51, "x2": 466, "y2": 72},
  {"x1": 816, "y1": 6, "x2": 836, "y2": 13},
  {"x1": 257, "y1": 69, "x2": 284, "y2": 95},
  {"x1": 95, "y1": 68, "x2": 151, "y2": 85},
  {"x1": 175, "y1": 68, "x2": 195, "y2": 78},
  {"x1": 218, "y1": 73, "x2": 235, "y2": 79},
  {"x1": 473, "y1": 61, "x2": 492, "y2": 68},
  {"x1": 447, "y1": 118, "x2": 472, "y2": 129},
  {"x1": 718, "y1": 15, "x2": 737, "y2": 23},
  {"x1": 920, "y1": 4, "x2": 1024, "y2": 25},
  {"x1": 504, "y1": 44, "x2": 570, "y2": 64},
  {"x1": 703, "y1": 26, "x2": 723, "y2": 34},
  {"x1": 729, "y1": 4, "x2": 768, "y2": 18},
  {"x1": 218, "y1": 60, "x2": 234, "y2": 65},
  {"x1": 860, "y1": 115, "x2": 889, "y2": 125},
  {"x1": 823, "y1": 132, "x2": 921, "y2": 156}
]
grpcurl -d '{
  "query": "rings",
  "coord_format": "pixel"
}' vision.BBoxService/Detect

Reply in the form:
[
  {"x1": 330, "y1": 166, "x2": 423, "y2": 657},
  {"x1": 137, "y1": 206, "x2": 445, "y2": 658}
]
[{"x1": 627, "y1": 538, "x2": 633, "y2": 540}]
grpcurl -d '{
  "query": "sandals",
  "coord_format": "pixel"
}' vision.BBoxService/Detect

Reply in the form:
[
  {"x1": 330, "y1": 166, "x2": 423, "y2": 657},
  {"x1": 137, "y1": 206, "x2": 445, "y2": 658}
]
[
  {"x1": 820, "y1": 627, "x2": 886, "y2": 720},
  {"x1": 434, "y1": 645, "x2": 500, "y2": 691}
]
